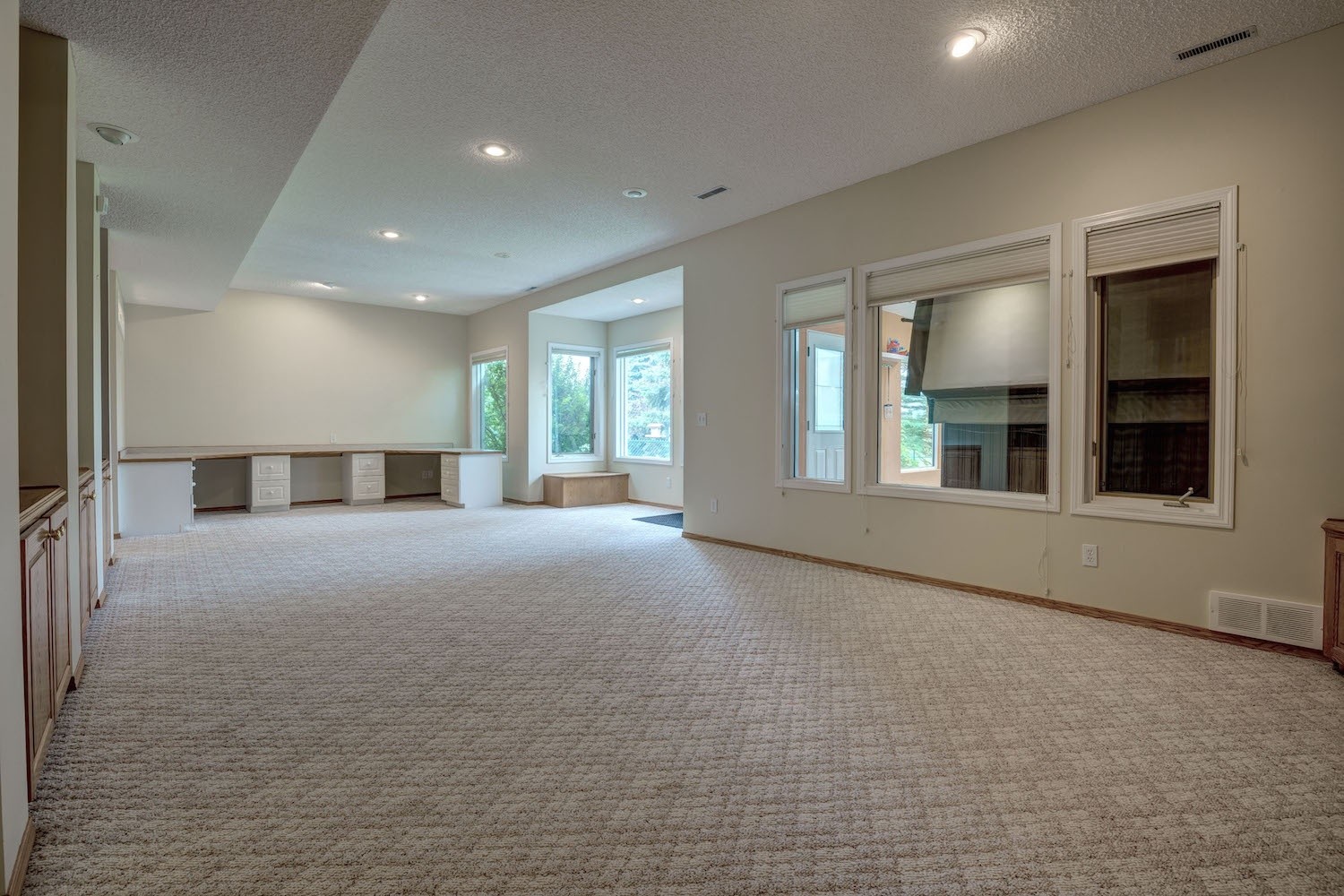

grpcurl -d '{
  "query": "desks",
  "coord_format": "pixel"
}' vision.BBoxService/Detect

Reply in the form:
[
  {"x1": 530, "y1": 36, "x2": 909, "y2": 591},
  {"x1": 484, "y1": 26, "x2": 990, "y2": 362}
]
[{"x1": 121, "y1": 442, "x2": 504, "y2": 462}]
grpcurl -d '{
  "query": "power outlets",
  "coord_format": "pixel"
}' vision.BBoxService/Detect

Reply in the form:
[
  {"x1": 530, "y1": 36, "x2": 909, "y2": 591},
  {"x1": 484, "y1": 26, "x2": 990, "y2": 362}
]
[
  {"x1": 666, "y1": 478, "x2": 672, "y2": 488},
  {"x1": 710, "y1": 498, "x2": 718, "y2": 513},
  {"x1": 330, "y1": 434, "x2": 336, "y2": 443},
  {"x1": 1081, "y1": 544, "x2": 1098, "y2": 567}
]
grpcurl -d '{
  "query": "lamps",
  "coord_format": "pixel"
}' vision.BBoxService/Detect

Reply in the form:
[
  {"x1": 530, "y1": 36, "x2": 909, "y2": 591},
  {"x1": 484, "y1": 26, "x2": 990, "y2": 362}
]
[
  {"x1": 940, "y1": 28, "x2": 985, "y2": 58},
  {"x1": 86, "y1": 123, "x2": 135, "y2": 147},
  {"x1": 322, "y1": 282, "x2": 334, "y2": 289}
]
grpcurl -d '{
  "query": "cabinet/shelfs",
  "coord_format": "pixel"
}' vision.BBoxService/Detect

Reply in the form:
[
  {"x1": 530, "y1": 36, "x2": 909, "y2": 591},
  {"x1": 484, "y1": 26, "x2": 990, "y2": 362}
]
[
  {"x1": 120, "y1": 460, "x2": 196, "y2": 536},
  {"x1": 1320, "y1": 515, "x2": 1344, "y2": 669},
  {"x1": 440, "y1": 454, "x2": 506, "y2": 510},
  {"x1": 244, "y1": 455, "x2": 292, "y2": 512},
  {"x1": 78, "y1": 466, "x2": 98, "y2": 643},
  {"x1": 103, "y1": 458, "x2": 112, "y2": 576},
  {"x1": 21, "y1": 502, "x2": 71, "y2": 803},
  {"x1": 342, "y1": 452, "x2": 389, "y2": 505}
]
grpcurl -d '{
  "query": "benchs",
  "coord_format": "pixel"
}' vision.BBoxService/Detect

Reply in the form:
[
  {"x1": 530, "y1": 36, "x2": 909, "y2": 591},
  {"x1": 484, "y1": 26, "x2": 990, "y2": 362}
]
[{"x1": 542, "y1": 471, "x2": 629, "y2": 508}]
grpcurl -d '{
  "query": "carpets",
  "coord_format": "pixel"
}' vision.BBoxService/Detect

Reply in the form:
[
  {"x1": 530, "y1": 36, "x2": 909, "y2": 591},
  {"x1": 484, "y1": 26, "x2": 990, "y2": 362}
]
[{"x1": 631, "y1": 512, "x2": 682, "y2": 530}]
